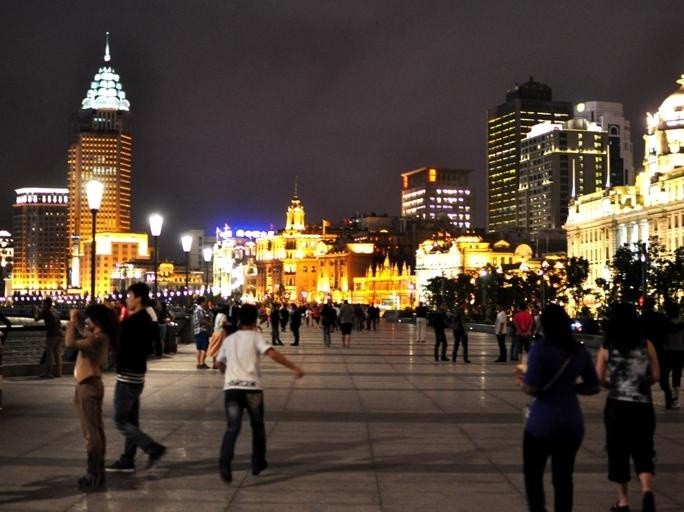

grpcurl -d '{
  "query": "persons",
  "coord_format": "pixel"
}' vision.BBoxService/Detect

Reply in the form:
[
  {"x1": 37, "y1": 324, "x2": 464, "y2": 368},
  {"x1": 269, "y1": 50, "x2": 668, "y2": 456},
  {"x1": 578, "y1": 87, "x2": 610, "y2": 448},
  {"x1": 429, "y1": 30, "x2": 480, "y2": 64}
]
[
  {"x1": 595, "y1": 302, "x2": 660, "y2": 512},
  {"x1": 572, "y1": 301, "x2": 683, "y2": 410},
  {"x1": 256, "y1": 302, "x2": 302, "y2": 346},
  {"x1": 411, "y1": 301, "x2": 498, "y2": 328},
  {"x1": 103, "y1": 283, "x2": 166, "y2": 473},
  {"x1": 494, "y1": 303, "x2": 508, "y2": 362},
  {"x1": 507, "y1": 302, "x2": 545, "y2": 361},
  {"x1": 215, "y1": 303, "x2": 304, "y2": 484},
  {"x1": 64, "y1": 303, "x2": 116, "y2": 492},
  {"x1": 452, "y1": 301, "x2": 472, "y2": 363},
  {"x1": 1, "y1": 288, "x2": 131, "y2": 378},
  {"x1": 414, "y1": 302, "x2": 427, "y2": 342},
  {"x1": 146, "y1": 296, "x2": 242, "y2": 369},
  {"x1": 512, "y1": 303, "x2": 603, "y2": 512},
  {"x1": 431, "y1": 302, "x2": 450, "y2": 361},
  {"x1": 299, "y1": 299, "x2": 380, "y2": 349}
]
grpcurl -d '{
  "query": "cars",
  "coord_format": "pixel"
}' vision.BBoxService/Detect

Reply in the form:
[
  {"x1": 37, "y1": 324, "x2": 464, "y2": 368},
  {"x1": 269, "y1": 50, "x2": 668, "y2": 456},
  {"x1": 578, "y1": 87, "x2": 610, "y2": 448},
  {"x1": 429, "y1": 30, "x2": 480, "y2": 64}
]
[
  {"x1": 381, "y1": 309, "x2": 412, "y2": 323},
  {"x1": 571, "y1": 317, "x2": 582, "y2": 332}
]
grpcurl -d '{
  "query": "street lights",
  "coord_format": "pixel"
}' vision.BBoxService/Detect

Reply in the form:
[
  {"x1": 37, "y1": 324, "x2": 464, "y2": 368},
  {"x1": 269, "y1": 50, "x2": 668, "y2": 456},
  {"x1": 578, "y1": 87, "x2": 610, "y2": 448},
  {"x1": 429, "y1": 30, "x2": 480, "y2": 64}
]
[
  {"x1": 147, "y1": 210, "x2": 163, "y2": 299},
  {"x1": 202, "y1": 245, "x2": 213, "y2": 293},
  {"x1": 83, "y1": 177, "x2": 105, "y2": 304},
  {"x1": 180, "y1": 235, "x2": 193, "y2": 294}
]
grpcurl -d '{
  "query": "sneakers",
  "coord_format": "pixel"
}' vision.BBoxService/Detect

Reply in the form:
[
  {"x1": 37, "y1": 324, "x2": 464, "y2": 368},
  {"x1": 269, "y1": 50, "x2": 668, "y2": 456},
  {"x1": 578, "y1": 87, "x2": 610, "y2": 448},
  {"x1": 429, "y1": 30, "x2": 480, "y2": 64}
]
[
  {"x1": 218, "y1": 454, "x2": 232, "y2": 483},
  {"x1": 104, "y1": 460, "x2": 136, "y2": 473},
  {"x1": 144, "y1": 445, "x2": 166, "y2": 470},
  {"x1": 672, "y1": 399, "x2": 680, "y2": 409},
  {"x1": 252, "y1": 461, "x2": 266, "y2": 476}
]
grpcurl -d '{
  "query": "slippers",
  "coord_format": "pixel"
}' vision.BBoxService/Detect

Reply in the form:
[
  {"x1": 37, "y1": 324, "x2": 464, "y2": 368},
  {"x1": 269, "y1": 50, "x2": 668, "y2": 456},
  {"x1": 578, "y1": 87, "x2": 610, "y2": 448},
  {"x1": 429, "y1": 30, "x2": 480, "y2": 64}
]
[
  {"x1": 642, "y1": 490, "x2": 655, "y2": 512},
  {"x1": 608, "y1": 500, "x2": 630, "y2": 512}
]
